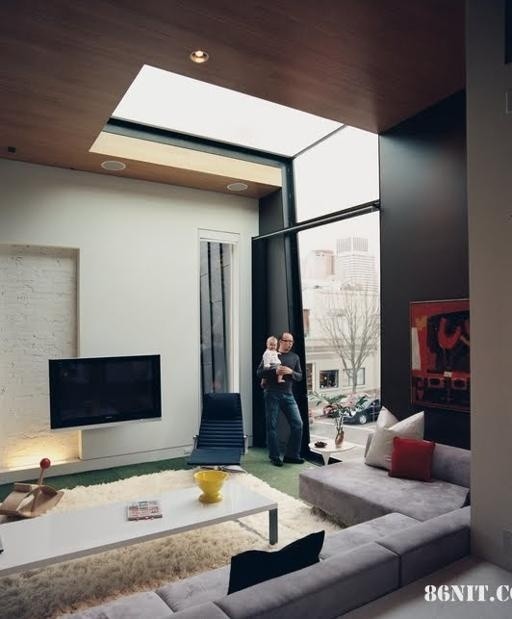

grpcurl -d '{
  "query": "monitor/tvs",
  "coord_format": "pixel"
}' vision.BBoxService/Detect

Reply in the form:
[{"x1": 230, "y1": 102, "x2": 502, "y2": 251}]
[{"x1": 48, "y1": 354, "x2": 163, "y2": 429}]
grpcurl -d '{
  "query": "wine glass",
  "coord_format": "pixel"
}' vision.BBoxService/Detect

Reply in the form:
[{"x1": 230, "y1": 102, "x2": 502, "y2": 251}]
[{"x1": 194, "y1": 469, "x2": 229, "y2": 504}]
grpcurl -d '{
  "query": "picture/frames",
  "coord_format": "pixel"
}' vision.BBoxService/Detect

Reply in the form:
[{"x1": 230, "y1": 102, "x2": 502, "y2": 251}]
[{"x1": 406, "y1": 293, "x2": 470, "y2": 418}]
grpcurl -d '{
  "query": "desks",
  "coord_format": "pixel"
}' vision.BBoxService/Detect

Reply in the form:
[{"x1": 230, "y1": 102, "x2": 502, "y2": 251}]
[
  {"x1": 307, "y1": 440, "x2": 356, "y2": 469},
  {"x1": 0, "y1": 481, "x2": 281, "y2": 579}
]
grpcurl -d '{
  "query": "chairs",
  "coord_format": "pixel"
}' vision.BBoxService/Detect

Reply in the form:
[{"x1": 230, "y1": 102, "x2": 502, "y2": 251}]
[{"x1": 186, "y1": 391, "x2": 251, "y2": 473}]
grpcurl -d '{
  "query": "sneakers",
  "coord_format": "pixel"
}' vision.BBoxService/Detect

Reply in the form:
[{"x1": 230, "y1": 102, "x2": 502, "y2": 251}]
[
  {"x1": 271, "y1": 458, "x2": 284, "y2": 467},
  {"x1": 283, "y1": 456, "x2": 306, "y2": 464}
]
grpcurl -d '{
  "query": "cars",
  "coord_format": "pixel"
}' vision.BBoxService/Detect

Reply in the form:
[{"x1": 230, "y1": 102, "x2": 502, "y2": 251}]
[
  {"x1": 324, "y1": 393, "x2": 370, "y2": 417},
  {"x1": 340, "y1": 396, "x2": 381, "y2": 425},
  {"x1": 307, "y1": 393, "x2": 328, "y2": 417}
]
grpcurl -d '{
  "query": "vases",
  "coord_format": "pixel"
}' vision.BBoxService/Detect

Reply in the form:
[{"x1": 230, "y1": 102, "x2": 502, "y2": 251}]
[{"x1": 334, "y1": 430, "x2": 346, "y2": 445}]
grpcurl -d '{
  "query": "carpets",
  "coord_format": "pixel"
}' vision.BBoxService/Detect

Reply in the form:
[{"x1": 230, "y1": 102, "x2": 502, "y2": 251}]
[{"x1": 0, "y1": 461, "x2": 349, "y2": 619}]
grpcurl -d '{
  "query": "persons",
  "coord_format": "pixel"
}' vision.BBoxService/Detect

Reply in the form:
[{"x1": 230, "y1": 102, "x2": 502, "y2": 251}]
[
  {"x1": 260, "y1": 335, "x2": 286, "y2": 388},
  {"x1": 257, "y1": 332, "x2": 304, "y2": 466}
]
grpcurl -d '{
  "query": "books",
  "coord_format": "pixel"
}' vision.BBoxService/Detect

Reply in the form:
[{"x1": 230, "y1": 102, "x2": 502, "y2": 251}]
[{"x1": 127, "y1": 499, "x2": 162, "y2": 520}]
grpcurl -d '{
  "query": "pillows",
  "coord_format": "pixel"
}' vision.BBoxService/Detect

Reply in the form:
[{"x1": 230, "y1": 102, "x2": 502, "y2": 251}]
[
  {"x1": 387, "y1": 434, "x2": 436, "y2": 485},
  {"x1": 363, "y1": 405, "x2": 427, "y2": 472},
  {"x1": 458, "y1": 488, "x2": 472, "y2": 509},
  {"x1": 224, "y1": 529, "x2": 327, "y2": 597}
]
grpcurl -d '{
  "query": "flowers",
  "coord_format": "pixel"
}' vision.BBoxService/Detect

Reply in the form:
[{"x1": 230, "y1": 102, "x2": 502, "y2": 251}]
[{"x1": 303, "y1": 389, "x2": 370, "y2": 434}]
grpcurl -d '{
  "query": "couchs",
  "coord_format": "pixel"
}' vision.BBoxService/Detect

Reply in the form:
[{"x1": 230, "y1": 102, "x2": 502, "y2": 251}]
[
  {"x1": 49, "y1": 507, "x2": 476, "y2": 619},
  {"x1": 295, "y1": 433, "x2": 472, "y2": 524}
]
